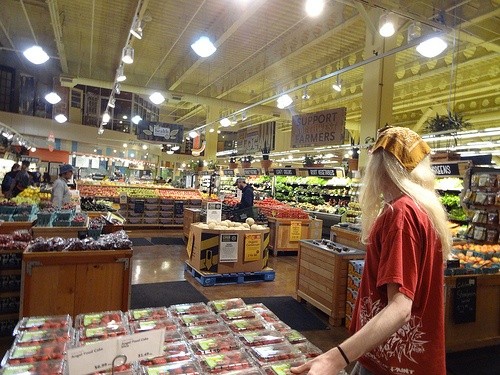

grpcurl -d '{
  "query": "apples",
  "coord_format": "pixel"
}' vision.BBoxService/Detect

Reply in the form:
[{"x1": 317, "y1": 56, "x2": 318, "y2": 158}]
[
  {"x1": 121, "y1": 187, "x2": 158, "y2": 198},
  {"x1": 0, "y1": 186, "x2": 51, "y2": 207},
  {"x1": 79, "y1": 185, "x2": 119, "y2": 197},
  {"x1": 160, "y1": 189, "x2": 201, "y2": 199},
  {"x1": 453, "y1": 243, "x2": 500, "y2": 269}
]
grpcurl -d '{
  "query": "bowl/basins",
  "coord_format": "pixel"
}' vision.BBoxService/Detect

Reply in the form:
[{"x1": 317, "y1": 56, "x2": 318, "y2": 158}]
[
  {"x1": 0, "y1": 206, "x2": 32, "y2": 221},
  {"x1": 37, "y1": 212, "x2": 87, "y2": 226},
  {"x1": 88, "y1": 226, "x2": 102, "y2": 237}
]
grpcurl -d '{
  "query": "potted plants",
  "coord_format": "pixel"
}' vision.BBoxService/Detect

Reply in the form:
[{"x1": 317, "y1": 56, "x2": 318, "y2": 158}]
[
  {"x1": 237, "y1": 155, "x2": 254, "y2": 168},
  {"x1": 261, "y1": 140, "x2": 273, "y2": 168},
  {"x1": 228, "y1": 158, "x2": 238, "y2": 169}
]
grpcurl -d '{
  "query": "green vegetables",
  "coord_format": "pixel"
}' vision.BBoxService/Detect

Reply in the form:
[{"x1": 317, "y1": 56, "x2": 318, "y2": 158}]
[
  {"x1": 435, "y1": 178, "x2": 466, "y2": 219},
  {"x1": 259, "y1": 174, "x2": 324, "y2": 205}
]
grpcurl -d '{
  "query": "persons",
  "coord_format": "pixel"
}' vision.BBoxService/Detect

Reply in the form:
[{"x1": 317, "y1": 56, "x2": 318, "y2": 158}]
[
  {"x1": 49, "y1": 163, "x2": 76, "y2": 209},
  {"x1": 1, "y1": 163, "x2": 20, "y2": 194},
  {"x1": 3, "y1": 160, "x2": 32, "y2": 197},
  {"x1": 289, "y1": 124, "x2": 454, "y2": 375},
  {"x1": 232, "y1": 177, "x2": 254, "y2": 220}
]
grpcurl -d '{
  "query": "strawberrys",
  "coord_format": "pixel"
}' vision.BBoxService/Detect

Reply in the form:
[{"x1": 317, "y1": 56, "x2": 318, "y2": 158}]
[{"x1": 8, "y1": 300, "x2": 321, "y2": 375}]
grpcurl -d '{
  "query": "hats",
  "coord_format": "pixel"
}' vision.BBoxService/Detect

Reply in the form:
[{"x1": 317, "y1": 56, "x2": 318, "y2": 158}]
[
  {"x1": 233, "y1": 177, "x2": 245, "y2": 185},
  {"x1": 59, "y1": 164, "x2": 74, "y2": 176}
]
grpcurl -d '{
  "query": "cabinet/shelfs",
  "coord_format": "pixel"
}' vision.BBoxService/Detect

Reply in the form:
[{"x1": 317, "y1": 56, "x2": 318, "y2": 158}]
[{"x1": 0, "y1": 173, "x2": 500, "y2": 351}]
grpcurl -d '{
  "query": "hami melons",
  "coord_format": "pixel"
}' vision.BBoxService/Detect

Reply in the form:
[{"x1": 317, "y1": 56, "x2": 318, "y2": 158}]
[{"x1": 194, "y1": 218, "x2": 266, "y2": 230}]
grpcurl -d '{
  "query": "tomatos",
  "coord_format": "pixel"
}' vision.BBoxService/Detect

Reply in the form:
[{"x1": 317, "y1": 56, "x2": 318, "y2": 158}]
[{"x1": 224, "y1": 197, "x2": 310, "y2": 220}]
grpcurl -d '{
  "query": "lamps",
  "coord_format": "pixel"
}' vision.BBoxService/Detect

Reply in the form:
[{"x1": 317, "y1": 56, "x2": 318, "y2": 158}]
[
  {"x1": 301, "y1": 86, "x2": 310, "y2": 100},
  {"x1": 277, "y1": 95, "x2": 293, "y2": 110},
  {"x1": 22, "y1": 46, "x2": 49, "y2": 65},
  {"x1": 2, "y1": 131, "x2": 36, "y2": 152},
  {"x1": 416, "y1": 37, "x2": 449, "y2": 58},
  {"x1": 45, "y1": 92, "x2": 65, "y2": 104},
  {"x1": 131, "y1": 115, "x2": 143, "y2": 125},
  {"x1": 98, "y1": 86, "x2": 120, "y2": 135},
  {"x1": 377, "y1": 11, "x2": 395, "y2": 37},
  {"x1": 190, "y1": 37, "x2": 221, "y2": 58},
  {"x1": 55, "y1": 114, "x2": 67, "y2": 124},
  {"x1": 332, "y1": 75, "x2": 343, "y2": 92},
  {"x1": 121, "y1": 45, "x2": 134, "y2": 64},
  {"x1": 149, "y1": 92, "x2": 167, "y2": 105},
  {"x1": 130, "y1": 19, "x2": 143, "y2": 41},
  {"x1": 116, "y1": 67, "x2": 128, "y2": 82}
]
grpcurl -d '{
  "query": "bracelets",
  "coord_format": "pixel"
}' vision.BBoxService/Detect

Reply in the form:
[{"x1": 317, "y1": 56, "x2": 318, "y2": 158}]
[{"x1": 335, "y1": 344, "x2": 351, "y2": 365}]
